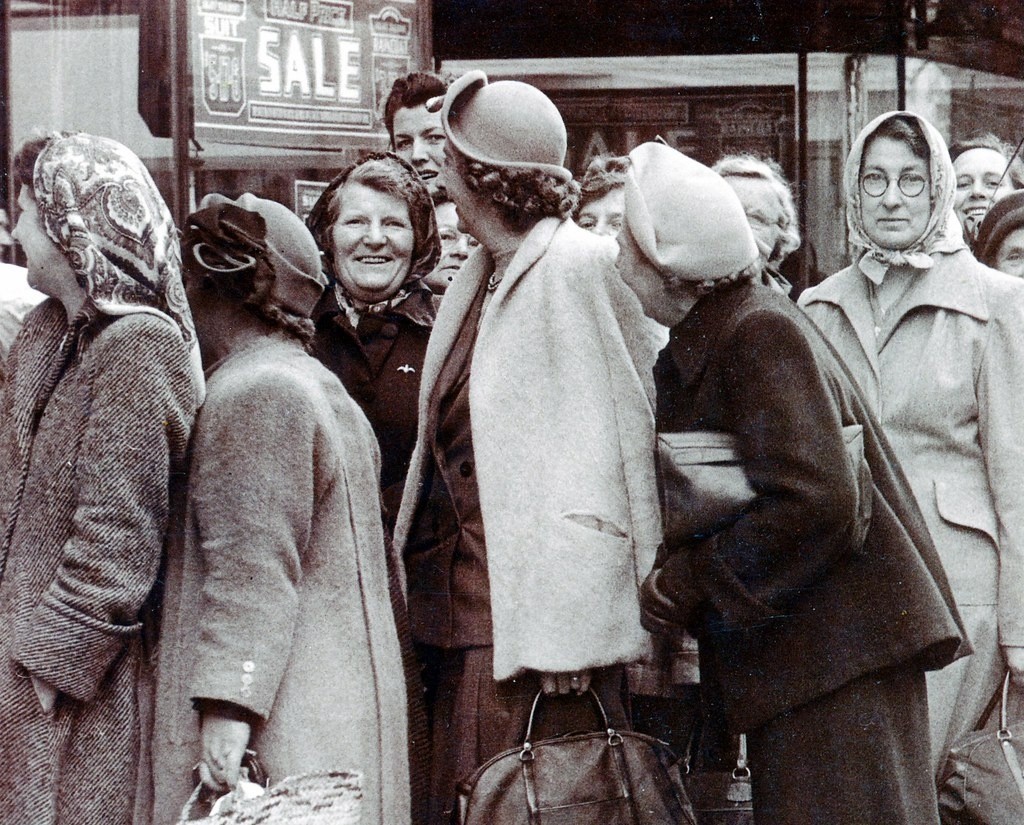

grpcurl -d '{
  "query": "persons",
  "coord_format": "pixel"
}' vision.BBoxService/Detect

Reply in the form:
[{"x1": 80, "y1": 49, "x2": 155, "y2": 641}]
[{"x1": 0, "y1": 70, "x2": 1024, "y2": 825}]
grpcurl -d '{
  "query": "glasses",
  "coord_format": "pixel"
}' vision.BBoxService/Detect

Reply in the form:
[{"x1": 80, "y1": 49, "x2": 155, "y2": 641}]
[{"x1": 862, "y1": 173, "x2": 927, "y2": 198}]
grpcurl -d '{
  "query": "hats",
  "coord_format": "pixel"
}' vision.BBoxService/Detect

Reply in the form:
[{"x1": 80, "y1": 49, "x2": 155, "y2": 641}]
[
  {"x1": 183, "y1": 192, "x2": 324, "y2": 321},
  {"x1": 623, "y1": 141, "x2": 760, "y2": 282},
  {"x1": 441, "y1": 69, "x2": 573, "y2": 184},
  {"x1": 974, "y1": 189, "x2": 1024, "y2": 267}
]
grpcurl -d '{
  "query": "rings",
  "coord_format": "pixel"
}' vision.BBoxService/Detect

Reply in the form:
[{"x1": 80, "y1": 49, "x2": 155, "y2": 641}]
[{"x1": 569, "y1": 677, "x2": 580, "y2": 680}]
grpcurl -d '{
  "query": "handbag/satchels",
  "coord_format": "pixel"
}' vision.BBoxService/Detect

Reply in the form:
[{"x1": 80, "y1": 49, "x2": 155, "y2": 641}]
[
  {"x1": 174, "y1": 765, "x2": 366, "y2": 825},
  {"x1": 677, "y1": 704, "x2": 754, "y2": 825},
  {"x1": 935, "y1": 669, "x2": 1024, "y2": 825},
  {"x1": 655, "y1": 425, "x2": 873, "y2": 556},
  {"x1": 456, "y1": 685, "x2": 699, "y2": 825}
]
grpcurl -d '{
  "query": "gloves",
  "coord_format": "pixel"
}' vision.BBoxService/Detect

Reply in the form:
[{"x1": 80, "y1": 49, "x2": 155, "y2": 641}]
[{"x1": 638, "y1": 569, "x2": 688, "y2": 653}]
